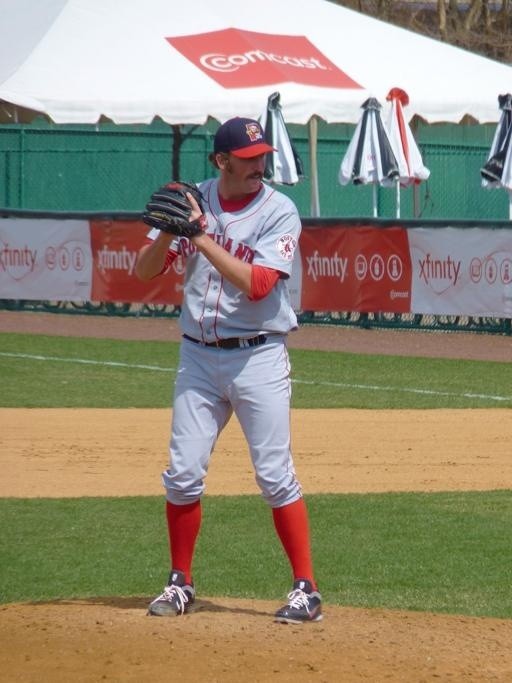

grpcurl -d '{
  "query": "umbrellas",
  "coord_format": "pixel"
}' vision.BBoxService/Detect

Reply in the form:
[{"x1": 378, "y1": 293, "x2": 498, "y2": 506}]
[
  {"x1": 337, "y1": 92, "x2": 401, "y2": 216},
  {"x1": 254, "y1": 89, "x2": 309, "y2": 186},
  {"x1": 385, "y1": 85, "x2": 431, "y2": 219},
  {"x1": 479, "y1": 90, "x2": 512, "y2": 220}
]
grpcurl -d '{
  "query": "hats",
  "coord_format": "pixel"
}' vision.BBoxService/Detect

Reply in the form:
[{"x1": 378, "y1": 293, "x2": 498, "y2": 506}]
[{"x1": 213, "y1": 117, "x2": 278, "y2": 159}]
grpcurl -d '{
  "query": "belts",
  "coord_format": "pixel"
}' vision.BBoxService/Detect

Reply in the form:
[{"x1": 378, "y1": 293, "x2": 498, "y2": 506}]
[{"x1": 182, "y1": 334, "x2": 267, "y2": 349}]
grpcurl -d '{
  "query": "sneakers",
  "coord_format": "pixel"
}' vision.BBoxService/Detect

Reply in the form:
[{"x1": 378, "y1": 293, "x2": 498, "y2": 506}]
[
  {"x1": 147, "y1": 570, "x2": 196, "y2": 617},
  {"x1": 274, "y1": 579, "x2": 322, "y2": 624}
]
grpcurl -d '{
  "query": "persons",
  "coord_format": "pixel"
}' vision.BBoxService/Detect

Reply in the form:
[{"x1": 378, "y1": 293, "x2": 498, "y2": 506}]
[{"x1": 133, "y1": 113, "x2": 325, "y2": 623}]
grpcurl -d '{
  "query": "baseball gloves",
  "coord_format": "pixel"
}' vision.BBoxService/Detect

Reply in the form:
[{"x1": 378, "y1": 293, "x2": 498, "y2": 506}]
[{"x1": 143, "y1": 179, "x2": 208, "y2": 236}]
